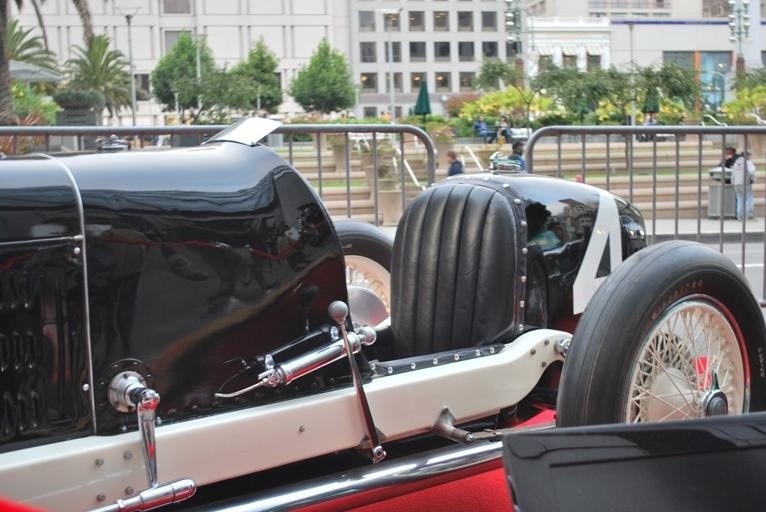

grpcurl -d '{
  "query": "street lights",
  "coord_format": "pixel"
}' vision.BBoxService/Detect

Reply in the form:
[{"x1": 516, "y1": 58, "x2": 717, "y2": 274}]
[
  {"x1": 505, "y1": 0, "x2": 534, "y2": 90},
  {"x1": 728, "y1": 0, "x2": 751, "y2": 113},
  {"x1": 188, "y1": 33, "x2": 207, "y2": 116},
  {"x1": 254, "y1": 85, "x2": 262, "y2": 109},
  {"x1": 111, "y1": 5, "x2": 142, "y2": 127},
  {"x1": 374, "y1": 8, "x2": 404, "y2": 144}
]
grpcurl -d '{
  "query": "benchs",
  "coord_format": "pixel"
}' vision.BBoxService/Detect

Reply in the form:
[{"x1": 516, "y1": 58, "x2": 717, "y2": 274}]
[{"x1": 474, "y1": 124, "x2": 511, "y2": 144}]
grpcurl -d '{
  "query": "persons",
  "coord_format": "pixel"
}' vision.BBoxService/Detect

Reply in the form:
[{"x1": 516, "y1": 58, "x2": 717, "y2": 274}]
[
  {"x1": 474, "y1": 118, "x2": 497, "y2": 143},
  {"x1": 732, "y1": 152, "x2": 759, "y2": 217},
  {"x1": 508, "y1": 141, "x2": 524, "y2": 170},
  {"x1": 446, "y1": 152, "x2": 466, "y2": 177},
  {"x1": 719, "y1": 145, "x2": 739, "y2": 167},
  {"x1": 497, "y1": 116, "x2": 517, "y2": 143}
]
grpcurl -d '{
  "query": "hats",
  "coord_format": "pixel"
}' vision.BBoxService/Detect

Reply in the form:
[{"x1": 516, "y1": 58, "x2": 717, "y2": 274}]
[{"x1": 512, "y1": 140, "x2": 526, "y2": 149}]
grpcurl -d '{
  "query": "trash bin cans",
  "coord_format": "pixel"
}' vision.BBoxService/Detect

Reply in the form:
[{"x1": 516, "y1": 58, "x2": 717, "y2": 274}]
[{"x1": 708, "y1": 166, "x2": 737, "y2": 220}]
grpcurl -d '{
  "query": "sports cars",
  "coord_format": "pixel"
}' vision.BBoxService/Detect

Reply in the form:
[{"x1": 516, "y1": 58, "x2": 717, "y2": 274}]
[{"x1": 0, "y1": 116, "x2": 766, "y2": 510}]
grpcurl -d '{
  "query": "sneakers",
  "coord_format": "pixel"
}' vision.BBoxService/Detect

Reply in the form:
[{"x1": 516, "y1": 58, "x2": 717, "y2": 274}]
[{"x1": 736, "y1": 214, "x2": 756, "y2": 223}]
